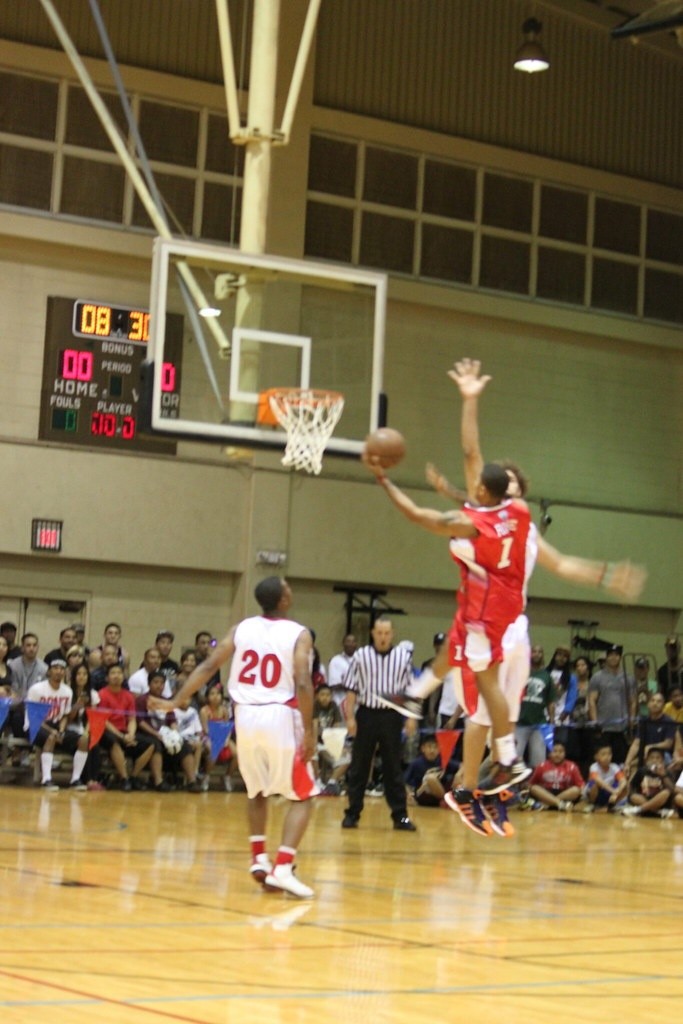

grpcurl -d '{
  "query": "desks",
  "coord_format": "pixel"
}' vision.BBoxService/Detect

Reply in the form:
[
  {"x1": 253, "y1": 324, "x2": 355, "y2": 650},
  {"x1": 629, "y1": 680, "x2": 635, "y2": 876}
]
[
  {"x1": 420, "y1": 727, "x2": 465, "y2": 762},
  {"x1": 554, "y1": 723, "x2": 599, "y2": 780}
]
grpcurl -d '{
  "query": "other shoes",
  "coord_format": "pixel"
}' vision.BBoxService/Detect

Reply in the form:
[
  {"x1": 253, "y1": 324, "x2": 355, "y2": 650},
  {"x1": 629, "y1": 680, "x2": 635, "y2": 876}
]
[
  {"x1": 155, "y1": 780, "x2": 176, "y2": 792},
  {"x1": 221, "y1": 774, "x2": 232, "y2": 792},
  {"x1": 659, "y1": 808, "x2": 675, "y2": 818},
  {"x1": 38, "y1": 778, "x2": 59, "y2": 791},
  {"x1": 620, "y1": 806, "x2": 638, "y2": 817},
  {"x1": 201, "y1": 774, "x2": 211, "y2": 791},
  {"x1": 187, "y1": 782, "x2": 203, "y2": 792},
  {"x1": 130, "y1": 776, "x2": 147, "y2": 790},
  {"x1": 68, "y1": 781, "x2": 87, "y2": 791},
  {"x1": 557, "y1": 801, "x2": 573, "y2": 812},
  {"x1": 393, "y1": 817, "x2": 416, "y2": 831},
  {"x1": 342, "y1": 805, "x2": 363, "y2": 827},
  {"x1": 119, "y1": 776, "x2": 132, "y2": 791}
]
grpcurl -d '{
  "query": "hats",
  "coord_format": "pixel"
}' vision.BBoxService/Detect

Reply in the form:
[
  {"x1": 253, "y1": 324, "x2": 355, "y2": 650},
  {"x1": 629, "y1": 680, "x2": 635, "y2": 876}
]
[
  {"x1": 606, "y1": 644, "x2": 623, "y2": 656},
  {"x1": 433, "y1": 632, "x2": 446, "y2": 645},
  {"x1": 555, "y1": 644, "x2": 571, "y2": 655}
]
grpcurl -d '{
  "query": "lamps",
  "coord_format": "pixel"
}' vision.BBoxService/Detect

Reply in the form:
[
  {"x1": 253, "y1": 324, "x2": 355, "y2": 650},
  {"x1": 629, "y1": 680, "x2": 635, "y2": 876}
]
[{"x1": 512, "y1": 18, "x2": 551, "y2": 73}]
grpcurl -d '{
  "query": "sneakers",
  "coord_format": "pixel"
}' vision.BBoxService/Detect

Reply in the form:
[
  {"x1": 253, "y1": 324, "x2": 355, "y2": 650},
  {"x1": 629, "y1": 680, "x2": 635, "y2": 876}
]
[
  {"x1": 444, "y1": 785, "x2": 492, "y2": 838},
  {"x1": 478, "y1": 789, "x2": 515, "y2": 838},
  {"x1": 265, "y1": 874, "x2": 312, "y2": 897},
  {"x1": 250, "y1": 860, "x2": 273, "y2": 885},
  {"x1": 477, "y1": 756, "x2": 533, "y2": 795}
]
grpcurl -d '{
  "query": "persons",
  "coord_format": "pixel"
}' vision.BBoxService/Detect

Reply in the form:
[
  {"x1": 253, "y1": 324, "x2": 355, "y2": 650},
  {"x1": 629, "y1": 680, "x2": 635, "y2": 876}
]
[
  {"x1": 342, "y1": 616, "x2": 418, "y2": 831},
  {"x1": 0, "y1": 620, "x2": 683, "y2": 823},
  {"x1": 444, "y1": 358, "x2": 647, "y2": 837},
  {"x1": 362, "y1": 452, "x2": 532, "y2": 800},
  {"x1": 147, "y1": 575, "x2": 327, "y2": 899}
]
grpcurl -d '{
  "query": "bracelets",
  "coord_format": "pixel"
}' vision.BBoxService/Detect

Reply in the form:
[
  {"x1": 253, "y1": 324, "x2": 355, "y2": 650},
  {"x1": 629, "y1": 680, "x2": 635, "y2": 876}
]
[
  {"x1": 377, "y1": 473, "x2": 390, "y2": 487},
  {"x1": 591, "y1": 560, "x2": 617, "y2": 592}
]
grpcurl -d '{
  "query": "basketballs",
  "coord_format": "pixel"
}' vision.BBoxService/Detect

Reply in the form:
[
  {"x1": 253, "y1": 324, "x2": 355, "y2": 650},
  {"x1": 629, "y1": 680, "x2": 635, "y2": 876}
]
[{"x1": 364, "y1": 427, "x2": 407, "y2": 468}]
